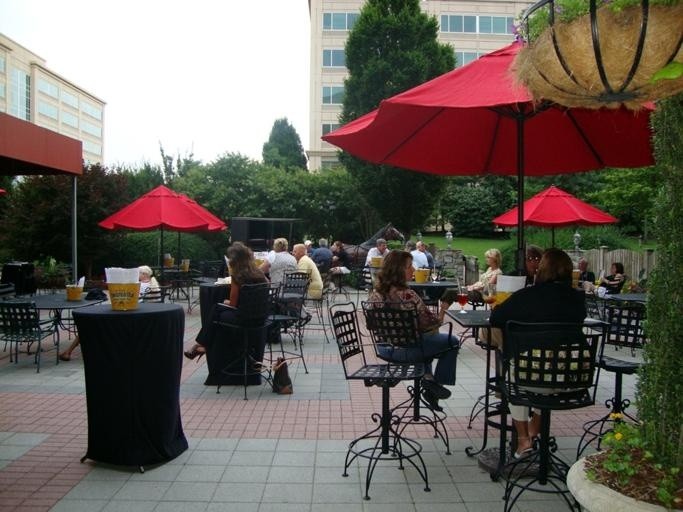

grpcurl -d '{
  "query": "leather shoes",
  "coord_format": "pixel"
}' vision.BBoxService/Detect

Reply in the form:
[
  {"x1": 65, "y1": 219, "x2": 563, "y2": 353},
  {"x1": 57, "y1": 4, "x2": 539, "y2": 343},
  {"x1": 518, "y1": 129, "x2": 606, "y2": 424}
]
[
  {"x1": 425, "y1": 394, "x2": 444, "y2": 412},
  {"x1": 422, "y1": 377, "x2": 451, "y2": 398}
]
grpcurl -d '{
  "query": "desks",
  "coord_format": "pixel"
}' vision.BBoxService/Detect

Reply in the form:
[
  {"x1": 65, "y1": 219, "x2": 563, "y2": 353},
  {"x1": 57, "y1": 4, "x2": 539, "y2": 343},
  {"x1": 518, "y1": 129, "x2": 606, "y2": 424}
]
[{"x1": 71, "y1": 303, "x2": 189, "y2": 474}]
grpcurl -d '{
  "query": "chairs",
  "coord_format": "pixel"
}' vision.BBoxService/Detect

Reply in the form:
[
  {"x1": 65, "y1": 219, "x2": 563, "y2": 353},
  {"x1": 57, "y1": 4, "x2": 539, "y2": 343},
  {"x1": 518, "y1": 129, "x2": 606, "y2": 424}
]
[
  {"x1": 142, "y1": 283, "x2": 173, "y2": 303},
  {"x1": 301, "y1": 255, "x2": 337, "y2": 344},
  {"x1": 329, "y1": 301, "x2": 432, "y2": 501},
  {"x1": 0, "y1": 302, "x2": 60, "y2": 374},
  {"x1": 360, "y1": 300, "x2": 461, "y2": 456},
  {"x1": 467, "y1": 325, "x2": 505, "y2": 430},
  {"x1": 212, "y1": 281, "x2": 282, "y2": 401},
  {"x1": 575, "y1": 292, "x2": 653, "y2": 461},
  {"x1": 265, "y1": 267, "x2": 314, "y2": 382},
  {"x1": 352, "y1": 245, "x2": 380, "y2": 315},
  {"x1": 500, "y1": 318, "x2": 608, "y2": 512}
]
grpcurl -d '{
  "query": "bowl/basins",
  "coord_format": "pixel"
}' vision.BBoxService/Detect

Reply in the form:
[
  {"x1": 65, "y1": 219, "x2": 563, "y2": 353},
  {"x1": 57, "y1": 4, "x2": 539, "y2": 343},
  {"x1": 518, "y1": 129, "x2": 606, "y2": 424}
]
[
  {"x1": 66, "y1": 285, "x2": 83, "y2": 301},
  {"x1": 106, "y1": 282, "x2": 142, "y2": 311},
  {"x1": 414, "y1": 269, "x2": 429, "y2": 283}
]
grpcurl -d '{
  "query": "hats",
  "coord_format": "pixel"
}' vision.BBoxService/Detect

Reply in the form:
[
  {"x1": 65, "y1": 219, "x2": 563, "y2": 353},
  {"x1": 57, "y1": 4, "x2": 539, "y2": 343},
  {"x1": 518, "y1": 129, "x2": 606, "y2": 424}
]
[
  {"x1": 86, "y1": 288, "x2": 108, "y2": 301},
  {"x1": 305, "y1": 240, "x2": 314, "y2": 246}
]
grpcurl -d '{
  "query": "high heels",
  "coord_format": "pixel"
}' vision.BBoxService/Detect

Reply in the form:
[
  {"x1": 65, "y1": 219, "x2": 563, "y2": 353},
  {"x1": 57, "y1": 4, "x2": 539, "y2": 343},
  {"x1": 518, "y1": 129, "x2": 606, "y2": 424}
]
[
  {"x1": 184, "y1": 344, "x2": 206, "y2": 363},
  {"x1": 529, "y1": 432, "x2": 538, "y2": 447},
  {"x1": 514, "y1": 438, "x2": 534, "y2": 459}
]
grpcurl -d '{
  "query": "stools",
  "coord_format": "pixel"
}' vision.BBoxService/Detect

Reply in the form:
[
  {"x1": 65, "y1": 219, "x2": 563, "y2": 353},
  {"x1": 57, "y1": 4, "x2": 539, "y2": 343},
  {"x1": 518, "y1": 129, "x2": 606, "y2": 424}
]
[
  {"x1": 330, "y1": 272, "x2": 352, "y2": 304},
  {"x1": 456, "y1": 289, "x2": 486, "y2": 351}
]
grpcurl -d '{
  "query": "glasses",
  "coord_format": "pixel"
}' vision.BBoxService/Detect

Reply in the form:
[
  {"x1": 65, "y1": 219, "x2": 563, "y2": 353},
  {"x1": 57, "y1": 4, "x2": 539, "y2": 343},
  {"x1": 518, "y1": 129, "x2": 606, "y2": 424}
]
[{"x1": 526, "y1": 255, "x2": 542, "y2": 261}]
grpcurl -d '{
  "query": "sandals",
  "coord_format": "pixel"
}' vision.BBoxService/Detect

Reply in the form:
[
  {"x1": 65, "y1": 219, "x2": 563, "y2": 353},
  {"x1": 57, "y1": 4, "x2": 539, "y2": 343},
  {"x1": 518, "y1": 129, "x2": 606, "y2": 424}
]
[{"x1": 59, "y1": 351, "x2": 71, "y2": 361}]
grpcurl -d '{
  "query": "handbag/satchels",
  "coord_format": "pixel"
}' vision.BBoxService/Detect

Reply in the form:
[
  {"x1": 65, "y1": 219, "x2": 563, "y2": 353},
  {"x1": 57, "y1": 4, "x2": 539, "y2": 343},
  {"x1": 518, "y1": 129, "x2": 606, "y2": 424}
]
[{"x1": 272, "y1": 357, "x2": 294, "y2": 395}]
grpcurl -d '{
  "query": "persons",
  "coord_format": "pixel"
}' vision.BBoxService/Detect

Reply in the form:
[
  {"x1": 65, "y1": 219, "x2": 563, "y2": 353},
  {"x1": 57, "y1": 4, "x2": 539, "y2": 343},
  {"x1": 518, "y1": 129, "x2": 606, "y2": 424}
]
[
  {"x1": 416, "y1": 241, "x2": 434, "y2": 271},
  {"x1": 405, "y1": 240, "x2": 429, "y2": 270},
  {"x1": 490, "y1": 246, "x2": 590, "y2": 461},
  {"x1": 364, "y1": 249, "x2": 460, "y2": 412},
  {"x1": 137, "y1": 265, "x2": 159, "y2": 300},
  {"x1": 363, "y1": 238, "x2": 391, "y2": 283},
  {"x1": 184, "y1": 242, "x2": 270, "y2": 364},
  {"x1": 330, "y1": 240, "x2": 352, "y2": 274},
  {"x1": 58, "y1": 334, "x2": 81, "y2": 361},
  {"x1": 577, "y1": 258, "x2": 596, "y2": 288},
  {"x1": 598, "y1": 262, "x2": 625, "y2": 295},
  {"x1": 292, "y1": 243, "x2": 324, "y2": 327},
  {"x1": 507, "y1": 243, "x2": 545, "y2": 288},
  {"x1": 304, "y1": 239, "x2": 316, "y2": 257},
  {"x1": 258, "y1": 237, "x2": 305, "y2": 299},
  {"x1": 311, "y1": 238, "x2": 333, "y2": 273},
  {"x1": 436, "y1": 248, "x2": 502, "y2": 323}
]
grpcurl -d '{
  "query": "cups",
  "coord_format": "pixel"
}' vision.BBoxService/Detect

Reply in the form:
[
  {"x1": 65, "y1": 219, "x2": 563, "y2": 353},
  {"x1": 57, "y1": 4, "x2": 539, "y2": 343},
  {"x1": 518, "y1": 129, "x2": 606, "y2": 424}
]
[
  {"x1": 482, "y1": 284, "x2": 498, "y2": 321},
  {"x1": 457, "y1": 286, "x2": 469, "y2": 314}
]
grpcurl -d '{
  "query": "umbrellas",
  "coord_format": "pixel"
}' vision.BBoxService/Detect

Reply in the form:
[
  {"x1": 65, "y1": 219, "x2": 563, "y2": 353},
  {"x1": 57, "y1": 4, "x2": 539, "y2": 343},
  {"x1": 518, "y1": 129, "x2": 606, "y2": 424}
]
[
  {"x1": 321, "y1": 37, "x2": 656, "y2": 273},
  {"x1": 492, "y1": 184, "x2": 619, "y2": 247},
  {"x1": 98, "y1": 184, "x2": 228, "y2": 282}
]
